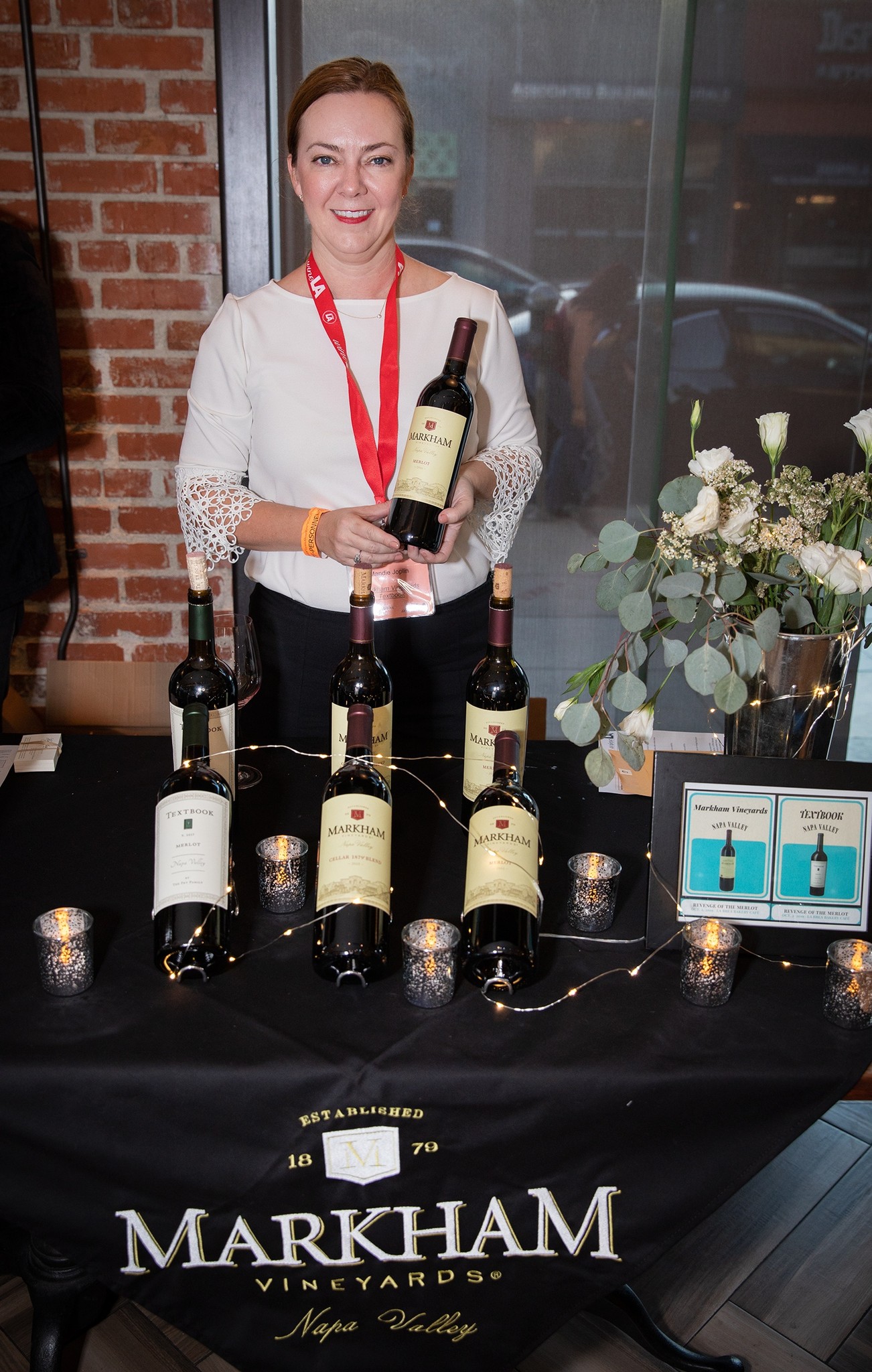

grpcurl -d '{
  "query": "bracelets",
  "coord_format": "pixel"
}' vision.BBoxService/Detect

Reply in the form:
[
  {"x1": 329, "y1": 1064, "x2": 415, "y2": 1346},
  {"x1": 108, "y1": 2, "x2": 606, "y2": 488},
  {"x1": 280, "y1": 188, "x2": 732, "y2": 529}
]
[{"x1": 300, "y1": 507, "x2": 329, "y2": 559}]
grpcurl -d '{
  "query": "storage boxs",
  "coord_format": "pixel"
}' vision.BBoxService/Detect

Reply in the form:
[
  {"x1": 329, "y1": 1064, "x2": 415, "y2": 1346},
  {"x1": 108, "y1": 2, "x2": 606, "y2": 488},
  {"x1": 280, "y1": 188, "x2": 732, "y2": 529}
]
[{"x1": 0, "y1": 659, "x2": 184, "y2": 735}]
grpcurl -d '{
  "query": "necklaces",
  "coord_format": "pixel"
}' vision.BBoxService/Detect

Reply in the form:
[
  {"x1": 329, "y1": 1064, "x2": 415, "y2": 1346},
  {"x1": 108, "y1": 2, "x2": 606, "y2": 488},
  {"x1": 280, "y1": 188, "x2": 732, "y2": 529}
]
[{"x1": 334, "y1": 300, "x2": 386, "y2": 319}]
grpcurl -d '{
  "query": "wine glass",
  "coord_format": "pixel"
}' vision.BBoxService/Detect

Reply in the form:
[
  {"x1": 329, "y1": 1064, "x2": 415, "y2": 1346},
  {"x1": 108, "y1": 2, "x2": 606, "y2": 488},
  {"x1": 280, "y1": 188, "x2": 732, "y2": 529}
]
[{"x1": 214, "y1": 615, "x2": 263, "y2": 789}]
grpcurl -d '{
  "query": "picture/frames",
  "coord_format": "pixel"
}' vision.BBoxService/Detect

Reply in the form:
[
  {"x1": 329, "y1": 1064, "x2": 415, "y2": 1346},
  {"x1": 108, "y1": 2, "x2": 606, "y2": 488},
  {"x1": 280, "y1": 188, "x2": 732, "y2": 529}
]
[{"x1": 645, "y1": 750, "x2": 872, "y2": 957}]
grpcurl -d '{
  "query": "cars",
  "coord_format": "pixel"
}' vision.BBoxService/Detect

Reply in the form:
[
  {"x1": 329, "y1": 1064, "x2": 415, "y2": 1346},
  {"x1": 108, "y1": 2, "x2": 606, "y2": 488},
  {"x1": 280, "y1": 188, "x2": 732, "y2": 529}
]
[
  {"x1": 395, "y1": 235, "x2": 548, "y2": 314},
  {"x1": 507, "y1": 279, "x2": 870, "y2": 513}
]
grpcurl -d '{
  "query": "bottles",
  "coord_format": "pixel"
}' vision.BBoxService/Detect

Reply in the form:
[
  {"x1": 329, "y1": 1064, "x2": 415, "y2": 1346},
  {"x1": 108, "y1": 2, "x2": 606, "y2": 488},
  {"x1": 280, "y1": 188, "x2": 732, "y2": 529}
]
[
  {"x1": 719, "y1": 829, "x2": 736, "y2": 891},
  {"x1": 312, "y1": 563, "x2": 392, "y2": 983},
  {"x1": 150, "y1": 551, "x2": 237, "y2": 981},
  {"x1": 809, "y1": 832, "x2": 827, "y2": 895},
  {"x1": 383, "y1": 317, "x2": 477, "y2": 553},
  {"x1": 462, "y1": 563, "x2": 539, "y2": 994}
]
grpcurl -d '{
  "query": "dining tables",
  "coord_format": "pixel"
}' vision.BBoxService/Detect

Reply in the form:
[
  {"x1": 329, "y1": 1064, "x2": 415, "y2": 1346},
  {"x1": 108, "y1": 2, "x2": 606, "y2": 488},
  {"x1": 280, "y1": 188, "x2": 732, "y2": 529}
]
[{"x1": 0, "y1": 717, "x2": 872, "y2": 1372}]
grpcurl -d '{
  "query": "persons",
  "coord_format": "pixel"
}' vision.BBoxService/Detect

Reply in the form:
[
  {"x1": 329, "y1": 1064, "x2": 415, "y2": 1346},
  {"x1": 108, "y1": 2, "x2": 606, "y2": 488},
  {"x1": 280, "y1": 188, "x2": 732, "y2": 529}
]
[
  {"x1": 538, "y1": 261, "x2": 638, "y2": 516},
  {"x1": 513, "y1": 281, "x2": 565, "y2": 502},
  {"x1": 167, "y1": 56, "x2": 546, "y2": 751}
]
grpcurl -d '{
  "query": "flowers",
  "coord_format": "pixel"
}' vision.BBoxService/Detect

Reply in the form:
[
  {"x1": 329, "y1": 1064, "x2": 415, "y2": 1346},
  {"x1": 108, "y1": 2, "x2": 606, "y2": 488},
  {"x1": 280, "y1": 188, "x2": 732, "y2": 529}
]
[{"x1": 553, "y1": 397, "x2": 872, "y2": 795}]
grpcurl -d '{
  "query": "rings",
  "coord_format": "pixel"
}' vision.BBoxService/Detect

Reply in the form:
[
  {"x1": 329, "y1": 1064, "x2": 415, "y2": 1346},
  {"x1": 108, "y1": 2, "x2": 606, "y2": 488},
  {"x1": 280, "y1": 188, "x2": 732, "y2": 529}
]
[{"x1": 353, "y1": 550, "x2": 362, "y2": 563}]
